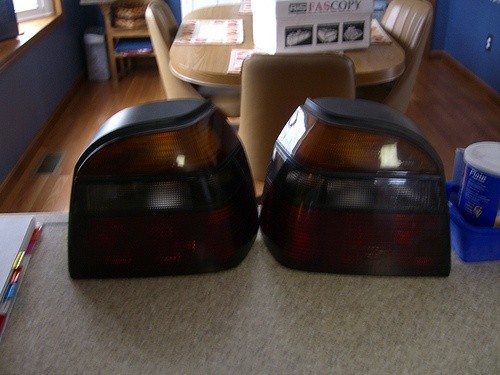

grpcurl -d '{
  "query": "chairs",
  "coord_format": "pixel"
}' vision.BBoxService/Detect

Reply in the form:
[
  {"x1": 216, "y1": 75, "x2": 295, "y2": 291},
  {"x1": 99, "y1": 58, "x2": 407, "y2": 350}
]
[
  {"x1": 354, "y1": 0, "x2": 433, "y2": 115},
  {"x1": 144, "y1": 0, "x2": 238, "y2": 126},
  {"x1": 239, "y1": 53, "x2": 355, "y2": 204}
]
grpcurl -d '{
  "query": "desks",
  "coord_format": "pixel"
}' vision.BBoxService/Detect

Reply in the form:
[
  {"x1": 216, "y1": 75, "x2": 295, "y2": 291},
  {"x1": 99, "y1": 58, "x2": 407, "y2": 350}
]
[
  {"x1": 170, "y1": 1, "x2": 405, "y2": 87},
  {"x1": 1, "y1": 206, "x2": 499, "y2": 375}
]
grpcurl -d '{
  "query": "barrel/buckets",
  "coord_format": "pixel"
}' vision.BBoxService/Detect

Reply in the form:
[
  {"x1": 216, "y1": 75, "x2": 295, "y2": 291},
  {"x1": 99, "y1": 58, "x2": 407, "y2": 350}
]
[{"x1": 457, "y1": 141, "x2": 500, "y2": 226}]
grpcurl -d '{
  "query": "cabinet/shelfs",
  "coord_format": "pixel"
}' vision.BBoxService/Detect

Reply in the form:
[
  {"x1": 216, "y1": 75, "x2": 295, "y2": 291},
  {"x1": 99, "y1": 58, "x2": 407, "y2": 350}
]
[{"x1": 101, "y1": 0, "x2": 156, "y2": 81}]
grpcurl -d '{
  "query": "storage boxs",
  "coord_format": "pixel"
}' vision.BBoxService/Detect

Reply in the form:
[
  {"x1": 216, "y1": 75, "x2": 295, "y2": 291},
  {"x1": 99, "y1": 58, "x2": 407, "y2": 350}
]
[
  {"x1": 445, "y1": 182, "x2": 500, "y2": 262},
  {"x1": 250, "y1": 1, "x2": 372, "y2": 53}
]
